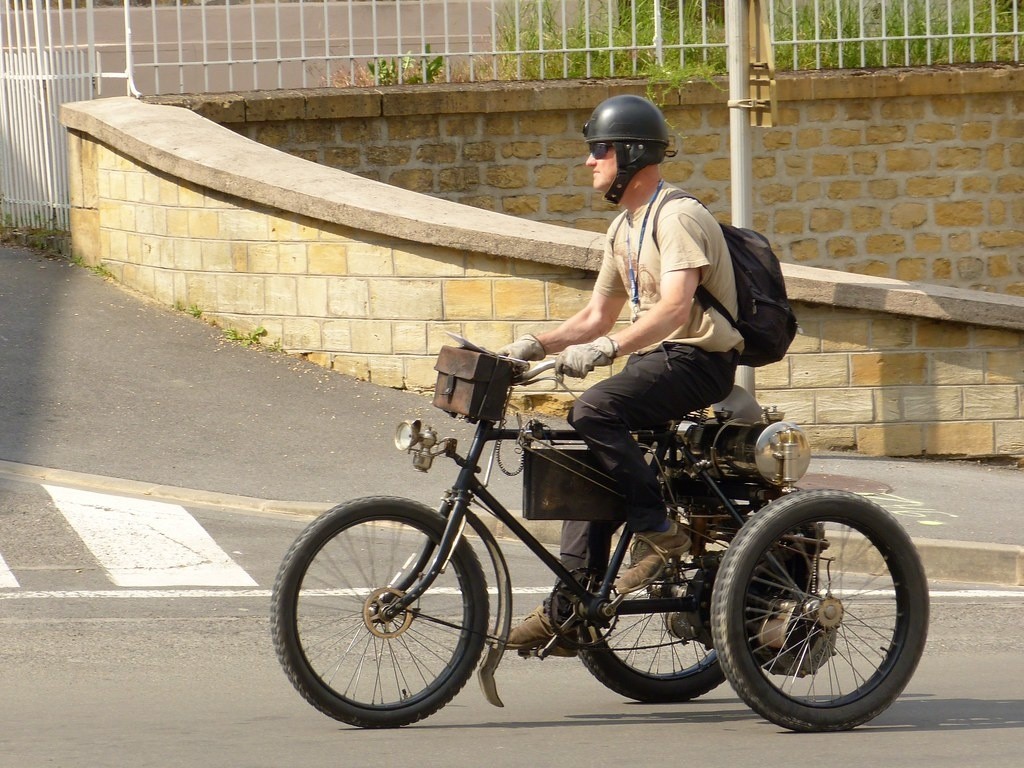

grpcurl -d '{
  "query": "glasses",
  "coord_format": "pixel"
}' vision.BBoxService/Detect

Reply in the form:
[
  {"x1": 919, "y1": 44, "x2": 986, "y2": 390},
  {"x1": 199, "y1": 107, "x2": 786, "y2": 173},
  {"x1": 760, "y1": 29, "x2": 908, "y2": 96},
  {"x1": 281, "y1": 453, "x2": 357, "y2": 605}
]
[{"x1": 589, "y1": 142, "x2": 614, "y2": 159}]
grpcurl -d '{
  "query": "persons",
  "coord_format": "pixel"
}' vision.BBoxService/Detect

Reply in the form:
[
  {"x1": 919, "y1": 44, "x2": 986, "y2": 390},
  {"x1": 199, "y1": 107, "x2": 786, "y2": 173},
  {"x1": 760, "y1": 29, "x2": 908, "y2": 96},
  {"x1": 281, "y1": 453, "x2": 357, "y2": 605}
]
[{"x1": 493, "y1": 93, "x2": 744, "y2": 659}]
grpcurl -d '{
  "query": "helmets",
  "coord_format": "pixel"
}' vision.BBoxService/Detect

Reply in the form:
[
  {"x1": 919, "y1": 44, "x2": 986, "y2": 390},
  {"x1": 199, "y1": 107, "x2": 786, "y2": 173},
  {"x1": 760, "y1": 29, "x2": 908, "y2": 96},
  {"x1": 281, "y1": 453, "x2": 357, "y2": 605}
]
[{"x1": 583, "y1": 94, "x2": 669, "y2": 147}]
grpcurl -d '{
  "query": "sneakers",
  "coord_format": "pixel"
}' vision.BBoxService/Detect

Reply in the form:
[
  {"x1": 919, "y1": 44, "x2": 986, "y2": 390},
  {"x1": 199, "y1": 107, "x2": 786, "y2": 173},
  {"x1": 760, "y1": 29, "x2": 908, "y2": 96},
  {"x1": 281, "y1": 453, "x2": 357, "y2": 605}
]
[
  {"x1": 485, "y1": 605, "x2": 578, "y2": 658},
  {"x1": 616, "y1": 518, "x2": 692, "y2": 592}
]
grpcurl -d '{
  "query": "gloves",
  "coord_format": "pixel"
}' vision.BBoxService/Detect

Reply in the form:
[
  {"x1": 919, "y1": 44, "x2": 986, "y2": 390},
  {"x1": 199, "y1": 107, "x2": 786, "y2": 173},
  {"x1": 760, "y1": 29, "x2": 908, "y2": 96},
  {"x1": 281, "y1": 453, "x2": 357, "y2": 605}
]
[
  {"x1": 555, "y1": 335, "x2": 617, "y2": 384},
  {"x1": 496, "y1": 333, "x2": 546, "y2": 362}
]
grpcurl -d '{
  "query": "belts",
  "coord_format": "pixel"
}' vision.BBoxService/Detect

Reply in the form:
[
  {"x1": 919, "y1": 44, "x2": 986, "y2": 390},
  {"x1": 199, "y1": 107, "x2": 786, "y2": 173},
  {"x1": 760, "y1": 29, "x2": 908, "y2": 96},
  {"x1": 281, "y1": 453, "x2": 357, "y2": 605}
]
[{"x1": 717, "y1": 347, "x2": 734, "y2": 365}]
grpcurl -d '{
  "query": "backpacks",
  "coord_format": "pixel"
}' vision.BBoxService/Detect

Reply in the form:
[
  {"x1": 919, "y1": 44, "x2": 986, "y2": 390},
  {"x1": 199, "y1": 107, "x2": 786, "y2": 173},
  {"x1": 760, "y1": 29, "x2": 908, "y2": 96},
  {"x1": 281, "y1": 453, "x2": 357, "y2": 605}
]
[{"x1": 610, "y1": 189, "x2": 798, "y2": 368}]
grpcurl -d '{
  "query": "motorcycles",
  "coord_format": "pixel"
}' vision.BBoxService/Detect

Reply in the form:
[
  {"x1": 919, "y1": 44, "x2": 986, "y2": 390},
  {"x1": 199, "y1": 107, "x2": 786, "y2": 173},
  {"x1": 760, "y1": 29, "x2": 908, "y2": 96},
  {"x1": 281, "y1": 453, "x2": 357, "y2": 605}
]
[{"x1": 270, "y1": 331, "x2": 931, "y2": 740}]
